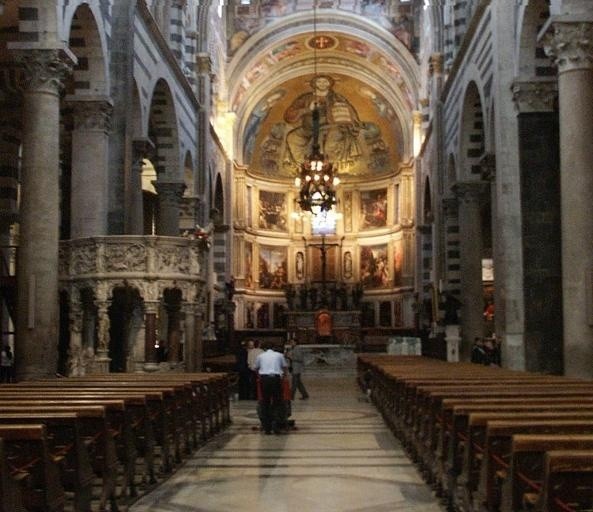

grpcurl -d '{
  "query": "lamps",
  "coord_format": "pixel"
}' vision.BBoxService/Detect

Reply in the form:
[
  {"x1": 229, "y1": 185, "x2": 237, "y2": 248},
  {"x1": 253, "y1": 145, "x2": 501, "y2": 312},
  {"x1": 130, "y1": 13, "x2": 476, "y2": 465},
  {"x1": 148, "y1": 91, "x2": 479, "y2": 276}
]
[{"x1": 293, "y1": 0, "x2": 341, "y2": 216}]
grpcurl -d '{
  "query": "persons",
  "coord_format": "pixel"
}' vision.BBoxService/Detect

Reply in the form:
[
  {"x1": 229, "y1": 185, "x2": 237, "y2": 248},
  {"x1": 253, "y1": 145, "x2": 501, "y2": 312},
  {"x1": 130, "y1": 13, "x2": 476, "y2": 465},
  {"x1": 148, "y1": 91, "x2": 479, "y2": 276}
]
[
  {"x1": 284, "y1": 74, "x2": 362, "y2": 164},
  {"x1": 238, "y1": 340, "x2": 265, "y2": 400},
  {"x1": 284, "y1": 338, "x2": 309, "y2": 400},
  {"x1": 484, "y1": 341, "x2": 498, "y2": 361},
  {"x1": 251, "y1": 341, "x2": 291, "y2": 435},
  {"x1": 470, "y1": 337, "x2": 485, "y2": 363}
]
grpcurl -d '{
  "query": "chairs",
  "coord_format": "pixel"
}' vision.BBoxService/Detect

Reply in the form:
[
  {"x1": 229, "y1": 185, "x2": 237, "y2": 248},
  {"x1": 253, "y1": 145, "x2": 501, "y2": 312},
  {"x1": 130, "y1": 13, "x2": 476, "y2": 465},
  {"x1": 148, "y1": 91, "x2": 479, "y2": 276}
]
[{"x1": 312, "y1": 309, "x2": 336, "y2": 345}]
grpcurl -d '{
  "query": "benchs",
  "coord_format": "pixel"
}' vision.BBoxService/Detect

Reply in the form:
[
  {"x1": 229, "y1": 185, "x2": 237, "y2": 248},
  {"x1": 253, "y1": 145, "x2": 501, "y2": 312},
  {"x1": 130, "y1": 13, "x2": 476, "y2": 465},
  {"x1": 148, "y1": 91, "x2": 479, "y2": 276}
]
[
  {"x1": 356, "y1": 353, "x2": 592, "y2": 512},
  {"x1": 0, "y1": 368, "x2": 240, "y2": 512}
]
